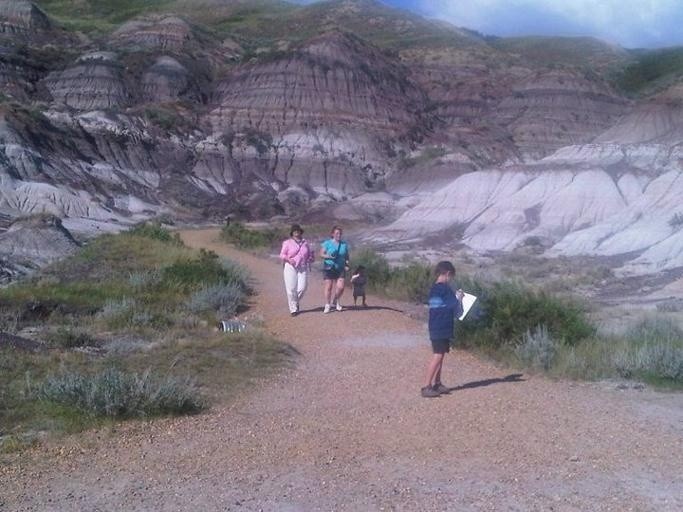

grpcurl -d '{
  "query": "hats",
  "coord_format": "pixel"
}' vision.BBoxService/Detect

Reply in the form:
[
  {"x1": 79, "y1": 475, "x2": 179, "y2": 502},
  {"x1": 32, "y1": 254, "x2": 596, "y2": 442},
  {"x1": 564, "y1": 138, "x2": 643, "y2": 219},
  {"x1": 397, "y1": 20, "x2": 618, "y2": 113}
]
[{"x1": 288, "y1": 224, "x2": 304, "y2": 237}]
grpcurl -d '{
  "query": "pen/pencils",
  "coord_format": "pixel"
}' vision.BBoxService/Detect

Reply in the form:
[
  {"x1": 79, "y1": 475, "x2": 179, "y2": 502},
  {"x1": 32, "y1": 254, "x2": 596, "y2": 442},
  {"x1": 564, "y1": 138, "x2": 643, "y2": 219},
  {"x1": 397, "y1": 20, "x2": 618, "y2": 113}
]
[{"x1": 456, "y1": 287, "x2": 466, "y2": 297}]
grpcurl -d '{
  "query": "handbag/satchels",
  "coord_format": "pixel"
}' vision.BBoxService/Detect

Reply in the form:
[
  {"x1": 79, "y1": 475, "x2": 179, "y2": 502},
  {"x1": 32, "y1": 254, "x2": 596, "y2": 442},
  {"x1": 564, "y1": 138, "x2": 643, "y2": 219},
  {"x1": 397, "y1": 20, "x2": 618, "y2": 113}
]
[{"x1": 323, "y1": 263, "x2": 337, "y2": 276}]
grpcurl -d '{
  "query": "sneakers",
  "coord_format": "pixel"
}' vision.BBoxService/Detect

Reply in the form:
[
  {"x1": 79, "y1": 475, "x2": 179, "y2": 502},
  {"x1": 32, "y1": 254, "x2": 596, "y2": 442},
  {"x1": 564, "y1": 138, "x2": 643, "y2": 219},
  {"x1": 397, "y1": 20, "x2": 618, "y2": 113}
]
[
  {"x1": 332, "y1": 299, "x2": 343, "y2": 311},
  {"x1": 419, "y1": 383, "x2": 441, "y2": 398},
  {"x1": 433, "y1": 384, "x2": 450, "y2": 394},
  {"x1": 323, "y1": 303, "x2": 333, "y2": 313}
]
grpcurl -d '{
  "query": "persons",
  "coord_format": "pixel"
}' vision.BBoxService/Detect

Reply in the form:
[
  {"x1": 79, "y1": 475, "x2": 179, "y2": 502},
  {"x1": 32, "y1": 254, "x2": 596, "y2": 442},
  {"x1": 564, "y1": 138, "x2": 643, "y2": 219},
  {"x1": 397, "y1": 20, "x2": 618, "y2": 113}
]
[
  {"x1": 420, "y1": 261, "x2": 465, "y2": 399},
  {"x1": 351, "y1": 267, "x2": 368, "y2": 307},
  {"x1": 319, "y1": 226, "x2": 350, "y2": 313},
  {"x1": 280, "y1": 225, "x2": 316, "y2": 317}
]
